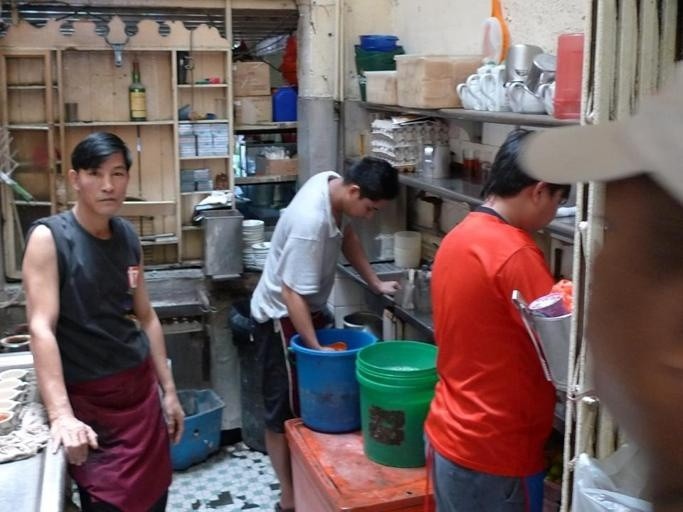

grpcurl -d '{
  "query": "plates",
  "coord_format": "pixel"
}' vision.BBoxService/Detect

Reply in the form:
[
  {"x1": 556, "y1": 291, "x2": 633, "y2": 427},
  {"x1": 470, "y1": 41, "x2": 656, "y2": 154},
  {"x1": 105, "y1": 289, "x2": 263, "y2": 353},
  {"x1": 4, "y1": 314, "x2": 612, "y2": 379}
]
[
  {"x1": 241, "y1": 219, "x2": 271, "y2": 272},
  {"x1": 0, "y1": 332, "x2": 32, "y2": 348},
  {"x1": 0, "y1": 368, "x2": 28, "y2": 426}
]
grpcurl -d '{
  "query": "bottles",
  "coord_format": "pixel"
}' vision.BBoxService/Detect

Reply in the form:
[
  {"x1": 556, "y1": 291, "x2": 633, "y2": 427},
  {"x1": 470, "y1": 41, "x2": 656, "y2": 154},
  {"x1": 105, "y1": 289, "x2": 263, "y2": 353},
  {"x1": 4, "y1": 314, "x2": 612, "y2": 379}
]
[
  {"x1": 177, "y1": 51, "x2": 189, "y2": 85},
  {"x1": 482, "y1": 1, "x2": 509, "y2": 64},
  {"x1": 127, "y1": 61, "x2": 147, "y2": 123}
]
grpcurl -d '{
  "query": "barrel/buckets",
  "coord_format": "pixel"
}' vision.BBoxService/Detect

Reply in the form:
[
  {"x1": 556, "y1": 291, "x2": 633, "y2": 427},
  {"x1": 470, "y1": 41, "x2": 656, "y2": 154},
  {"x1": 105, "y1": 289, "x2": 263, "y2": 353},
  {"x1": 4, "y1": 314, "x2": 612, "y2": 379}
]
[
  {"x1": 354, "y1": 44, "x2": 406, "y2": 102},
  {"x1": 271, "y1": 84, "x2": 298, "y2": 122},
  {"x1": 357, "y1": 34, "x2": 400, "y2": 53},
  {"x1": 287, "y1": 327, "x2": 376, "y2": 435},
  {"x1": 355, "y1": 338, "x2": 438, "y2": 471}
]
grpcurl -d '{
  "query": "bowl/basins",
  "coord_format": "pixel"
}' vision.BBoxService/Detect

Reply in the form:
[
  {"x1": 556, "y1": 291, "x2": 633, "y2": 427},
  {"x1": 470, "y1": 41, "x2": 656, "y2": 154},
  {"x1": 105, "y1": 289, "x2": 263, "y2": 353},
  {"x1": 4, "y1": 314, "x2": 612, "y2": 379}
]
[
  {"x1": 340, "y1": 310, "x2": 382, "y2": 339},
  {"x1": 357, "y1": 36, "x2": 399, "y2": 53}
]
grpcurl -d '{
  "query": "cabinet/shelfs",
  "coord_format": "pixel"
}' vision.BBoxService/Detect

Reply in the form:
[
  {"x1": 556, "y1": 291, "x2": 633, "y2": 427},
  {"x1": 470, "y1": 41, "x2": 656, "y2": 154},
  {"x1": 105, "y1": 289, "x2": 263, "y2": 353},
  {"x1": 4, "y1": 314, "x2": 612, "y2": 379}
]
[
  {"x1": 233, "y1": 120, "x2": 303, "y2": 190},
  {"x1": 331, "y1": 104, "x2": 582, "y2": 439},
  {"x1": 0, "y1": 17, "x2": 238, "y2": 278}
]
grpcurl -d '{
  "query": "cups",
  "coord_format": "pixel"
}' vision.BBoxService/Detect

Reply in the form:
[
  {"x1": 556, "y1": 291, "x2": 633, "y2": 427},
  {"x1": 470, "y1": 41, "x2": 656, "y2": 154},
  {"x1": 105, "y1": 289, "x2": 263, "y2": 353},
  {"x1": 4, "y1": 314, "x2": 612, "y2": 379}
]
[
  {"x1": 455, "y1": 66, "x2": 555, "y2": 116},
  {"x1": 506, "y1": 45, "x2": 541, "y2": 84},
  {"x1": 64, "y1": 103, "x2": 77, "y2": 122},
  {"x1": 461, "y1": 147, "x2": 481, "y2": 178},
  {"x1": 527, "y1": 293, "x2": 565, "y2": 321},
  {"x1": 523, "y1": 54, "x2": 555, "y2": 98}
]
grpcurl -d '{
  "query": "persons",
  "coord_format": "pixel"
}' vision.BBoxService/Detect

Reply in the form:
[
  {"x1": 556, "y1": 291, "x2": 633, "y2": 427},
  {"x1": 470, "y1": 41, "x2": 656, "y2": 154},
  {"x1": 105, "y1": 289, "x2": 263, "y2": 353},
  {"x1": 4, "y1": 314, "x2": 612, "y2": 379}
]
[
  {"x1": 248, "y1": 155, "x2": 402, "y2": 510},
  {"x1": 516, "y1": 81, "x2": 683, "y2": 510},
  {"x1": 424, "y1": 127, "x2": 570, "y2": 512},
  {"x1": 20, "y1": 131, "x2": 185, "y2": 512}
]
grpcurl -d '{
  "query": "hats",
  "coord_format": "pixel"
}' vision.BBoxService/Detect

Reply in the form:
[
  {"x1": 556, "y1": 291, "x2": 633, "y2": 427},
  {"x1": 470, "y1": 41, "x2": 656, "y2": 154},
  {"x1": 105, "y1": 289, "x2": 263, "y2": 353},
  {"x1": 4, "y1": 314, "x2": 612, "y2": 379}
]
[{"x1": 515, "y1": 55, "x2": 683, "y2": 210}]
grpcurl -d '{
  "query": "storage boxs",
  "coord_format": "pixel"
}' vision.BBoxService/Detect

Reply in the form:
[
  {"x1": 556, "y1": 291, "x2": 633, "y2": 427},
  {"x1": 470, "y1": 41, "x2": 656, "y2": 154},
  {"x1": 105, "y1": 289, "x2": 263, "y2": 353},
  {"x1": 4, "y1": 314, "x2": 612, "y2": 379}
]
[
  {"x1": 362, "y1": 70, "x2": 399, "y2": 107},
  {"x1": 234, "y1": 94, "x2": 274, "y2": 125},
  {"x1": 231, "y1": 61, "x2": 272, "y2": 99},
  {"x1": 393, "y1": 56, "x2": 480, "y2": 108},
  {"x1": 168, "y1": 385, "x2": 229, "y2": 473},
  {"x1": 254, "y1": 153, "x2": 300, "y2": 179}
]
